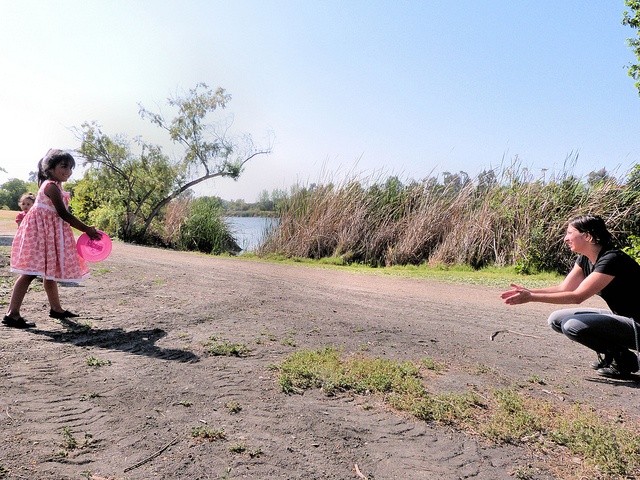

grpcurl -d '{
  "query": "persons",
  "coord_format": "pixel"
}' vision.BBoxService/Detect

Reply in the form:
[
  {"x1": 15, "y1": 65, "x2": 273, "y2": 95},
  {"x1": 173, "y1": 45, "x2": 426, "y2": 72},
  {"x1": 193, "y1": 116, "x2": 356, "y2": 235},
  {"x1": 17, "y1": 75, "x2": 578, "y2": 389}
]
[
  {"x1": 15, "y1": 192, "x2": 36, "y2": 229},
  {"x1": 1, "y1": 147, "x2": 103, "y2": 329},
  {"x1": 500, "y1": 214, "x2": 640, "y2": 380}
]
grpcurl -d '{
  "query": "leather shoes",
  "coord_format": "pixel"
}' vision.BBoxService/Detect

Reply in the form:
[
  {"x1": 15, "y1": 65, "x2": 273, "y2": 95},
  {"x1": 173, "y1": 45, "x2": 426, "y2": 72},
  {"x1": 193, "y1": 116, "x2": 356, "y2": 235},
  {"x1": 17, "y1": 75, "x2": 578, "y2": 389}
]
[
  {"x1": 590, "y1": 360, "x2": 612, "y2": 369},
  {"x1": 2, "y1": 315, "x2": 35, "y2": 328},
  {"x1": 50, "y1": 309, "x2": 79, "y2": 319},
  {"x1": 598, "y1": 365, "x2": 632, "y2": 378}
]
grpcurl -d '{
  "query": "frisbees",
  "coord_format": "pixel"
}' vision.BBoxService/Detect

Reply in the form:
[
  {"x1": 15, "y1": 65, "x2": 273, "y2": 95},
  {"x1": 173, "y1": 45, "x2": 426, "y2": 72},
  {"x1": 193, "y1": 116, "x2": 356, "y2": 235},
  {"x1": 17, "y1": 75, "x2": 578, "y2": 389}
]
[{"x1": 76, "y1": 230, "x2": 112, "y2": 263}]
